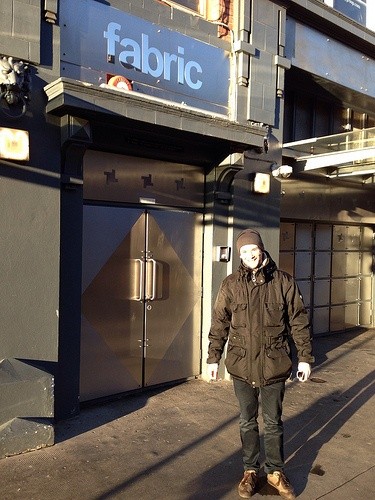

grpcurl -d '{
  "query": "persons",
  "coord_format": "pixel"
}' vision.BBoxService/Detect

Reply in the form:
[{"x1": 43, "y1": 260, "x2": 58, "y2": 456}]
[{"x1": 203, "y1": 229, "x2": 313, "y2": 500}]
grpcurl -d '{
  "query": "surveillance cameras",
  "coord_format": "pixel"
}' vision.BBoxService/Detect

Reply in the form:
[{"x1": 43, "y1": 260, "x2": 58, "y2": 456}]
[{"x1": 280, "y1": 165, "x2": 293, "y2": 178}]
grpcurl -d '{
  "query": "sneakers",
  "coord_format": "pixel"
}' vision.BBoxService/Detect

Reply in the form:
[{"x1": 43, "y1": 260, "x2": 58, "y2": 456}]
[
  {"x1": 267, "y1": 470, "x2": 297, "y2": 500},
  {"x1": 238, "y1": 470, "x2": 258, "y2": 498}
]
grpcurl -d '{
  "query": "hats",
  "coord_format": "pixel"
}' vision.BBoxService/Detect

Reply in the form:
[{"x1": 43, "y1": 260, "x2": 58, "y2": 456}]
[{"x1": 236, "y1": 228, "x2": 264, "y2": 255}]
